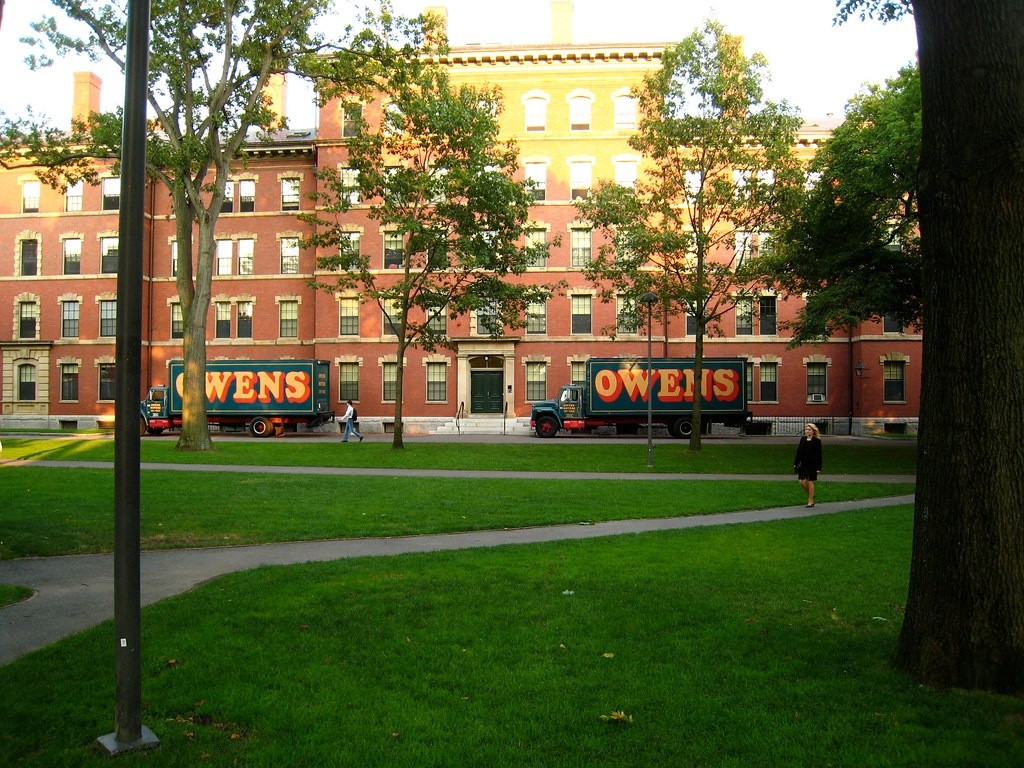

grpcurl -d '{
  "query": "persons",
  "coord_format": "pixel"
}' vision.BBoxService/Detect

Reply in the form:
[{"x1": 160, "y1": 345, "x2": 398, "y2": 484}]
[
  {"x1": 340, "y1": 399, "x2": 363, "y2": 442},
  {"x1": 794, "y1": 423, "x2": 823, "y2": 508}
]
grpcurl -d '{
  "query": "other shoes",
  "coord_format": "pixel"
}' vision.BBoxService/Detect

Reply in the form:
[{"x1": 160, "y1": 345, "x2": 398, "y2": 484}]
[
  {"x1": 359, "y1": 437, "x2": 363, "y2": 442},
  {"x1": 341, "y1": 440, "x2": 348, "y2": 442}
]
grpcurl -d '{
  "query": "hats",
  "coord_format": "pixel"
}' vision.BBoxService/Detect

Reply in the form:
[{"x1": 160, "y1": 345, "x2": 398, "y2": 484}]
[{"x1": 346, "y1": 400, "x2": 352, "y2": 403}]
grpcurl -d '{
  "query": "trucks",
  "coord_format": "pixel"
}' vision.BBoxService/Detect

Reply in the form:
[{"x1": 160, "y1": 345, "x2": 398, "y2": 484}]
[
  {"x1": 529, "y1": 356, "x2": 748, "y2": 439},
  {"x1": 139, "y1": 358, "x2": 335, "y2": 439}
]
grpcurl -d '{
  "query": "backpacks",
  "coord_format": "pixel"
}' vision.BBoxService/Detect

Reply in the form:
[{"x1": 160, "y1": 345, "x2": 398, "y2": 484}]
[{"x1": 353, "y1": 408, "x2": 357, "y2": 421}]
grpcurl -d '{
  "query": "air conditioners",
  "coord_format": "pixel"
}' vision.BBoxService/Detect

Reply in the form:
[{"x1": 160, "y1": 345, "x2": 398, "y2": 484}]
[{"x1": 812, "y1": 394, "x2": 822, "y2": 401}]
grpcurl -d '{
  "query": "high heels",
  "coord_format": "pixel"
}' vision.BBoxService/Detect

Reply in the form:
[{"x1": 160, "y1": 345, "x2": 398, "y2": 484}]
[{"x1": 806, "y1": 504, "x2": 814, "y2": 507}]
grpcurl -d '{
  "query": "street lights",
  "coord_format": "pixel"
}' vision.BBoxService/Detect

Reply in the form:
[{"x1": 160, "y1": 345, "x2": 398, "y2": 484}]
[{"x1": 640, "y1": 292, "x2": 659, "y2": 467}]
